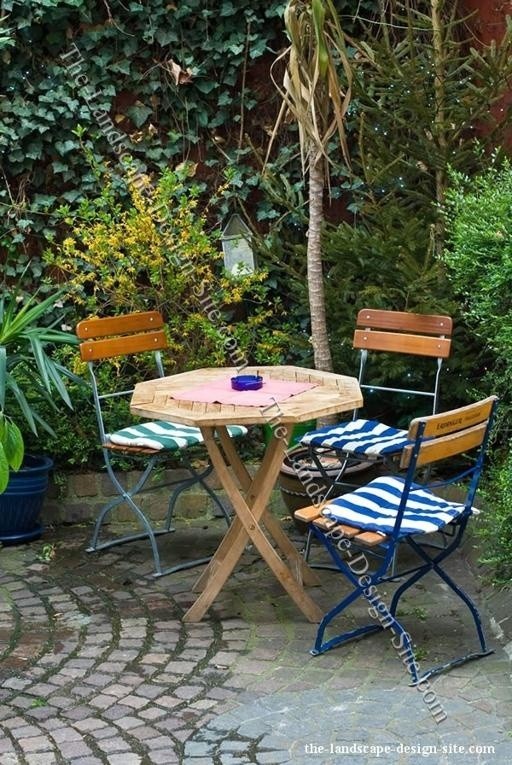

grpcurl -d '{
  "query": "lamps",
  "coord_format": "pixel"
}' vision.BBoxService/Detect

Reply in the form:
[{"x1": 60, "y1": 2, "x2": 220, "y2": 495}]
[{"x1": 217, "y1": 213, "x2": 255, "y2": 275}]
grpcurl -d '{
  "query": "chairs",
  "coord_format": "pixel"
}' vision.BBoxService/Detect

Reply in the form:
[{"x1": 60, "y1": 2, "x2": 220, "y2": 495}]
[
  {"x1": 299, "y1": 309, "x2": 454, "y2": 580},
  {"x1": 75, "y1": 311, "x2": 248, "y2": 579},
  {"x1": 295, "y1": 395, "x2": 499, "y2": 688}
]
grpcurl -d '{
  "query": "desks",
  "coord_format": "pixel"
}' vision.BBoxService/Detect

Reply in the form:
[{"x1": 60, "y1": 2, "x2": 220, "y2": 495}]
[{"x1": 130, "y1": 366, "x2": 365, "y2": 623}]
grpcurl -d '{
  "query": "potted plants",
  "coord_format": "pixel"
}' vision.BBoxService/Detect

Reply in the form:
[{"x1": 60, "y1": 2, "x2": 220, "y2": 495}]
[
  {"x1": 0, "y1": 257, "x2": 85, "y2": 547},
  {"x1": 260, "y1": 0, "x2": 383, "y2": 534}
]
